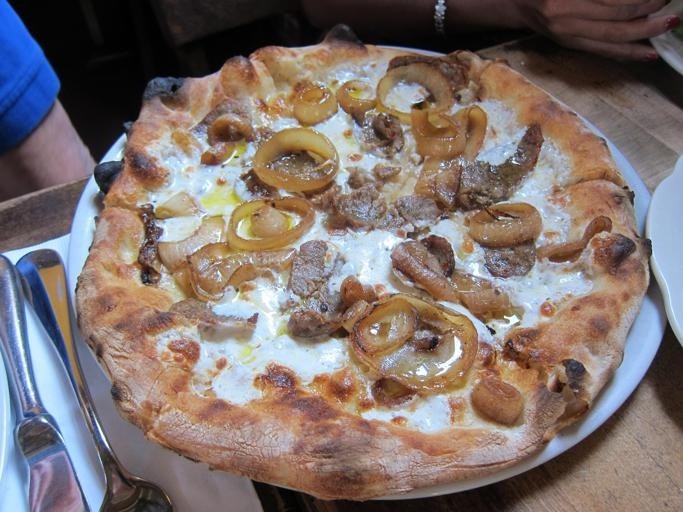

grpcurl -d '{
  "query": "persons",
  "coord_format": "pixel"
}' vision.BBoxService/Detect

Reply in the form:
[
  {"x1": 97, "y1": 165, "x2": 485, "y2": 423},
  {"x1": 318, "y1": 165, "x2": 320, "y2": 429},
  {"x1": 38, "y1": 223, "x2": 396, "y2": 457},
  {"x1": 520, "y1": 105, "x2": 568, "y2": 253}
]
[
  {"x1": 0, "y1": 0, "x2": 99, "y2": 203},
  {"x1": 303, "y1": 0, "x2": 682, "y2": 65}
]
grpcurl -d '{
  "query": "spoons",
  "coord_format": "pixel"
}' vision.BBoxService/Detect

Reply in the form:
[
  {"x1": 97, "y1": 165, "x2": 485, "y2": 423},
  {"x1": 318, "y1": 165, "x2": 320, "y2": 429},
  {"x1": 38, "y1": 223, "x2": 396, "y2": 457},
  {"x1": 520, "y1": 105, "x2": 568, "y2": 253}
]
[{"x1": 13, "y1": 248, "x2": 174, "y2": 512}]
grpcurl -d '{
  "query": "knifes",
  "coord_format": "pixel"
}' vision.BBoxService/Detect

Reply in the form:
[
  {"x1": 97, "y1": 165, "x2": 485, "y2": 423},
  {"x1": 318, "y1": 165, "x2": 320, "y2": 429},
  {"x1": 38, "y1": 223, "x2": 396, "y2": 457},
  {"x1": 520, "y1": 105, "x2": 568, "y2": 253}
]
[{"x1": 0, "y1": 252, "x2": 87, "y2": 512}]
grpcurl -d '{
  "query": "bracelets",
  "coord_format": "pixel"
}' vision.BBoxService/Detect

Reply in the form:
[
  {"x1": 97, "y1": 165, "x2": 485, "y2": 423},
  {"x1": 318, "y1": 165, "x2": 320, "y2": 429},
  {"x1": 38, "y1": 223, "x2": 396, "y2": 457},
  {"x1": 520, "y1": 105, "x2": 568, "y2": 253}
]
[{"x1": 430, "y1": 0, "x2": 452, "y2": 47}]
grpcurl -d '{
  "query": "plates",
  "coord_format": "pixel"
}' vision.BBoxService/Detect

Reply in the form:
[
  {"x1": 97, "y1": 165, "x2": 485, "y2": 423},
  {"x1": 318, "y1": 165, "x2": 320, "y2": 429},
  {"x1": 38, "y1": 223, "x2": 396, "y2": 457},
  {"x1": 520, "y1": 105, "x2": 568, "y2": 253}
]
[
  {"x1": 645, "y1": 1, "x2": 681, "y2": 74},
  {"x1": 643, "y1": 144, "x2": 683, "y2": 351}
]
